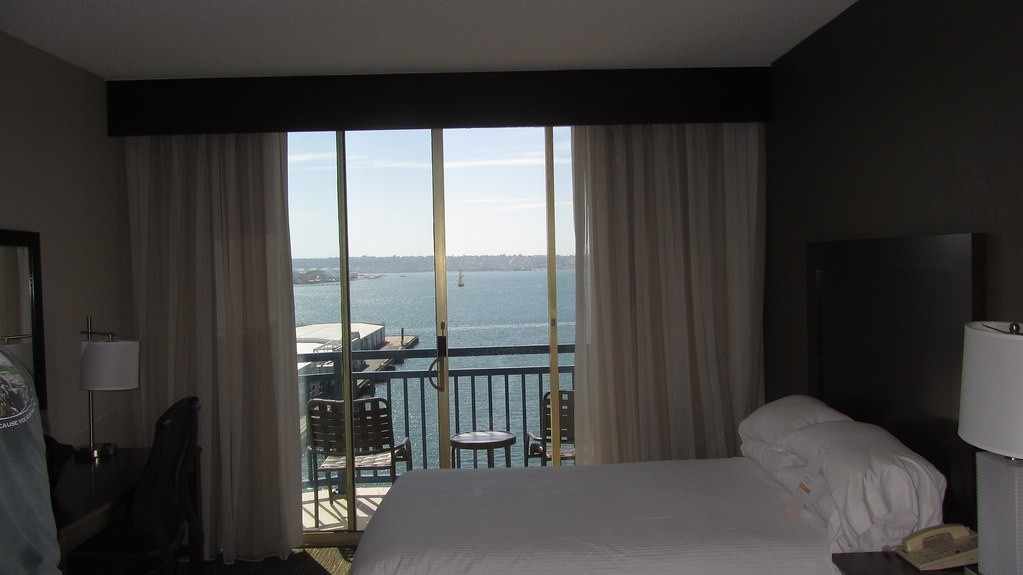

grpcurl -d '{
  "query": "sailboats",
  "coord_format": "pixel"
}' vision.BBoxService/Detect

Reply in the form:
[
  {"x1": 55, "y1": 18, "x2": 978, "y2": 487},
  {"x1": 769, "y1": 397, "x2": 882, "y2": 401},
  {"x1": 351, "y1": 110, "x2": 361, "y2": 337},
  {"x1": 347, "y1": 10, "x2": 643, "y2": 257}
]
[{"x1": 458, "y1": 270, "x2": 464, "y2": 287}]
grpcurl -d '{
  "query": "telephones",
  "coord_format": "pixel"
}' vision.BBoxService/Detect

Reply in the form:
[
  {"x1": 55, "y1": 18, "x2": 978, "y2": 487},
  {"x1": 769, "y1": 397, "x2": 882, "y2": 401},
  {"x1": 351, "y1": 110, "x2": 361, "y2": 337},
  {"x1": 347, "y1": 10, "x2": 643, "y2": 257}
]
[{"x1": 895, "y1": 524, "x2": 981, "y2": 571}]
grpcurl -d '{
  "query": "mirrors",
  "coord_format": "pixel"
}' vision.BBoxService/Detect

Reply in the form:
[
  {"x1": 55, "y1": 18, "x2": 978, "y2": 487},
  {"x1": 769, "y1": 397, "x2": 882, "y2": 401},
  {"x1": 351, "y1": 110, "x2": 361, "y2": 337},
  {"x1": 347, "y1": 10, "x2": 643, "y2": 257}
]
[{"x1": 0, "y1": 229, "x2": 48, "y2": 411}]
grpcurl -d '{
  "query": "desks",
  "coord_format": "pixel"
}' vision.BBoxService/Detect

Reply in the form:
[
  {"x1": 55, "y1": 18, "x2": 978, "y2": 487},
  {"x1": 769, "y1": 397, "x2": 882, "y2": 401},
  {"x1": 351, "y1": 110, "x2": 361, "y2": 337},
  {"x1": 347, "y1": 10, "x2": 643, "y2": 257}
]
[
  {"x1": 832, "y1": 548, "x2": 982, "y2": 575},
  {"x1": 449, "y1": 431, "x2": 516, "y2": 469},
  {"x1": 34, "y1": 444, "x2": 206, "y2": 575}
]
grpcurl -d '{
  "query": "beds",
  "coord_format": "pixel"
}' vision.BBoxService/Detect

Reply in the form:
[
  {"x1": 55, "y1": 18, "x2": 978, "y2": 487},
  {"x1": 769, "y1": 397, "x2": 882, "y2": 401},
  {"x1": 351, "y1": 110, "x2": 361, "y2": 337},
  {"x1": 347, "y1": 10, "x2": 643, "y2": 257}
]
[{"x1": 346, "y1": 396, "x2": 950, "y2": 575}]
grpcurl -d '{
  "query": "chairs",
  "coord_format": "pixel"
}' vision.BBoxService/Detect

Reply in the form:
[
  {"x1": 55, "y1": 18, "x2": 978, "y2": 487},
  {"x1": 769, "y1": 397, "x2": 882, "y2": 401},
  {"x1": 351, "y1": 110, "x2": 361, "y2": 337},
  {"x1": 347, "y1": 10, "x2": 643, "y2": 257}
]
[
  {"x1": 526, "y1": 389, "x2": 575, "y2": 466},
  {"x1": 66, "y1": 396, "x2": 207, "y2": 575},
  {"x1": 302, "y1": 395, "x2": 415, "y2": 529}
]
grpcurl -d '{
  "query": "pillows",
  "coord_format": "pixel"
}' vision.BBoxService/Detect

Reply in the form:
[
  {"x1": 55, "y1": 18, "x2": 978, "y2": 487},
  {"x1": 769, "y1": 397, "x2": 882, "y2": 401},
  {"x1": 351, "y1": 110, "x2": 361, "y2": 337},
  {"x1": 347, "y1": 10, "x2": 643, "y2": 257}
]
[{"x1": 737, "y1": 395, "x2": 947, "y2": 550}]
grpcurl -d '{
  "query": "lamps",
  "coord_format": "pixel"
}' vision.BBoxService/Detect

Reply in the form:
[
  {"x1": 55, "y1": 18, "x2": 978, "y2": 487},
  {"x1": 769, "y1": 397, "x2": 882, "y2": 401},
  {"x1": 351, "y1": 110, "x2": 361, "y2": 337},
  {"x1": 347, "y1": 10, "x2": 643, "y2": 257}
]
[
  {"x1": 958, "y1": 323, "x2": 1023, "y2": 575},
  {"x1": 78, "y1": 315, "x2": 139, "y2": 458}
]
